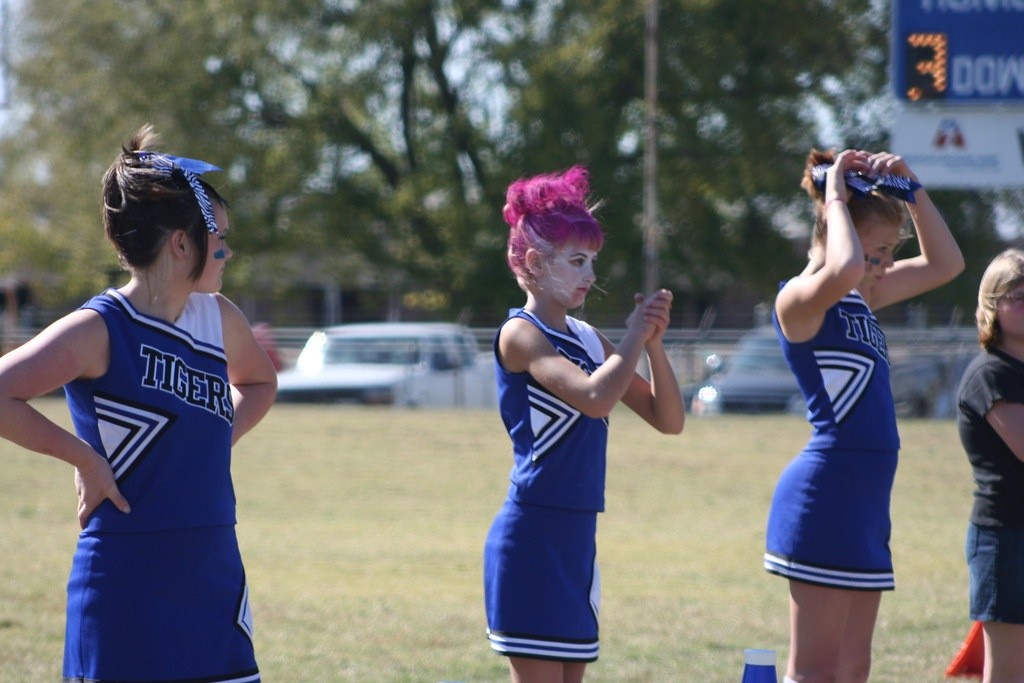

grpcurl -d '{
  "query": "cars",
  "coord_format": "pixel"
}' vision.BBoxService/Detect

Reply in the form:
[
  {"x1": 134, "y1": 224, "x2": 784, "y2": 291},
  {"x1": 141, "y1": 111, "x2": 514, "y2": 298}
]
[
  {"x1": 687, "y1": 332, "x2": 963, "y2": 421},
  {"x1": 266, "y1": 322, "x2": 501, "y2": 409}
]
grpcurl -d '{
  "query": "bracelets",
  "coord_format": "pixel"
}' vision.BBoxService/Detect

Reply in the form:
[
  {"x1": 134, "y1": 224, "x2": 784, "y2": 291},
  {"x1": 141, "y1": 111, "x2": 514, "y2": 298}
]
[{"x1": 826, "y1": 198, "x2": 845, "y2": 209}]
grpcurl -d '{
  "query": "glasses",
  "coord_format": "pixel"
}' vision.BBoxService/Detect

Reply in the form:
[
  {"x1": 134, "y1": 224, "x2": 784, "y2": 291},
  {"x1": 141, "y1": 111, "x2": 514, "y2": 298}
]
[{"x1": 999, "y1": 294, "x2": 1024, "y2": 307}]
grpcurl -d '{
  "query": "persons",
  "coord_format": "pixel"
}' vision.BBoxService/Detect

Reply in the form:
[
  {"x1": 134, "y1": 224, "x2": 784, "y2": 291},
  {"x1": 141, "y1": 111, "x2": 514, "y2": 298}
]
[
  {"x1": 485, "y1": 164, "x2": 684, "y2": 683},
  {"x1": 0, "y1": 124, "x2": 278, "y2": 683},
  {"x1": 765, "y1": 146, "x2": 966, "y2": 683},
  {"x1": 958, "y1": 247, "x2": 1024, "y2": 683}
]
